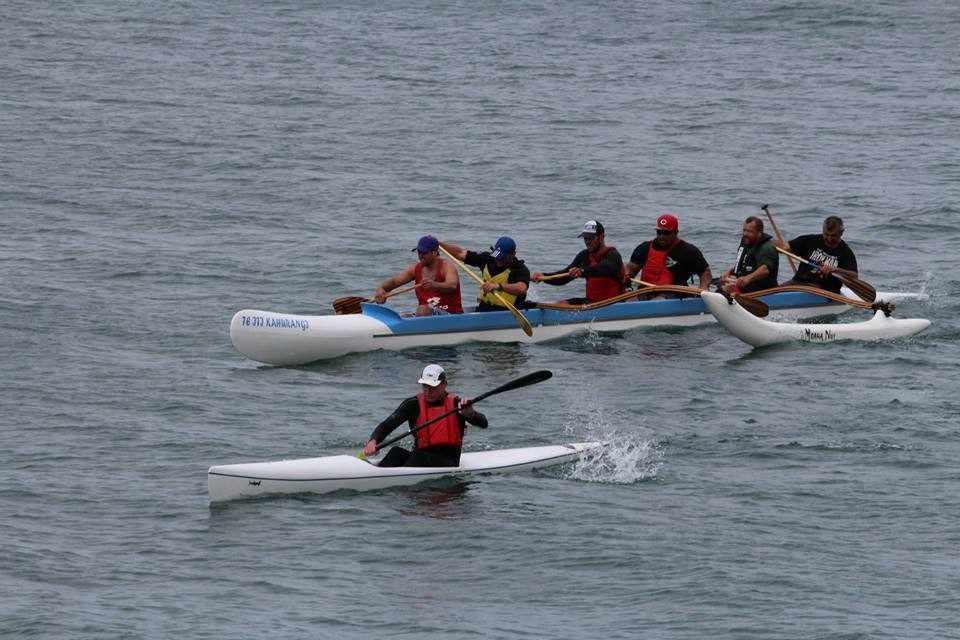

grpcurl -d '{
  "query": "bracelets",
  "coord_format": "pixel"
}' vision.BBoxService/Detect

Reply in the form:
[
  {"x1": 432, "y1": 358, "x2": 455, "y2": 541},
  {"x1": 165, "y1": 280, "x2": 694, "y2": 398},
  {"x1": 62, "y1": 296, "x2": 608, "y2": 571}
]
[{"x1": 496, "y1": 282, "x2": 503, "y2": 291}]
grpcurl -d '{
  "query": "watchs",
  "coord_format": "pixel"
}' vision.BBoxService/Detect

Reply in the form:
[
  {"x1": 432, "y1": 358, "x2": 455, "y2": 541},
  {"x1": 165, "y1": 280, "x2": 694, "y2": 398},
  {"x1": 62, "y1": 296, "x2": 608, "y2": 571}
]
[{"x1": 580, "y1": 267, "x2": 584, "y2": 275}]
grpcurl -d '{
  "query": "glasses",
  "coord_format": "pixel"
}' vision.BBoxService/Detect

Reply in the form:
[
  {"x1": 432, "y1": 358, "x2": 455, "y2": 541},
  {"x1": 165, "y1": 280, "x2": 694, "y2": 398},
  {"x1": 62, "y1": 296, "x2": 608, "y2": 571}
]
[{"x1": 657, "y1": 230, "x2": 675, "y2": 237}]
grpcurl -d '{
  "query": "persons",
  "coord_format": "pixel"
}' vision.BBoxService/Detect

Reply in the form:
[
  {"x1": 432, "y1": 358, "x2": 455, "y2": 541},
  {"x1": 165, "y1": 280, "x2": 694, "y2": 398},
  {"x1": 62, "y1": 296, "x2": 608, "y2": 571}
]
[
  {"x1": 440, "y1": 236, "x2": 530, "y2": 313},
  {"x1": 715, "y1": 217, "x2": 781, "y2": 299},
  {"x1": 623, "y1": 214, "x2": 712, "y2": 301},
  {"x1": 364, "y1": 364, "x2": 489, "y2": 468},
  {"x1": 529, "y1": 221, "x2": 625, "y2": 307},
  {"x1": 375, "y1": 235, "x2": 465, "y2": 318},
  {"x1": 770, "y1": 216, "x2": 859, "y2": 292}
]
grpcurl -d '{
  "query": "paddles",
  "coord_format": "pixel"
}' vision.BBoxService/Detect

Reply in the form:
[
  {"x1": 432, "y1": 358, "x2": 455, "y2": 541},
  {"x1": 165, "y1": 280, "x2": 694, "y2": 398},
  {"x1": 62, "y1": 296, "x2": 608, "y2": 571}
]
[
  {"x1": 332, "y1": 282, "x2": 426, "y2": 316},
  {"x1": 530, "y1": 271, "x2": 578, "y2": 281},
  {"x1": 438, "y1": 245, "x2": 534, "y2": 338},
  {"x1": 762, "y1": 204, "x2": 797, "y2": 274},
  {"x1": 361, "y1": 368, "x2": 554, "y2": 458},
  {"x1": 712, "y1": 277, "x2": 739, "y2": 286},
  {"x1": 623, "y1": 271, "x2": 769, "y2": 317},
  {"x1": 775, "y1": 246, "x2": 876, "y2": 303}
]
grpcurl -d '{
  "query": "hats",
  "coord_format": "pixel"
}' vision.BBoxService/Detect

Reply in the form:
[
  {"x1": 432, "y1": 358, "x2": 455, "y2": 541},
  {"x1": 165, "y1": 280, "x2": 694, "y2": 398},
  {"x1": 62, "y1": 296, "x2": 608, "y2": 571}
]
[
  {"x1": 418, "y1": 364, "x2": 447, "y2": 387},
  {"x1": 577, "y1": 220, "x2": 605, "y2": 238},
  {"x1": 654, "y1": 215, "x2": 678, "y2": 232},
  {"x1": 412, "y1": 234, "x2": 439, "y2": 253},
  {"x1": 489, "y1": 236, "x2": 517, "y2": 260}
]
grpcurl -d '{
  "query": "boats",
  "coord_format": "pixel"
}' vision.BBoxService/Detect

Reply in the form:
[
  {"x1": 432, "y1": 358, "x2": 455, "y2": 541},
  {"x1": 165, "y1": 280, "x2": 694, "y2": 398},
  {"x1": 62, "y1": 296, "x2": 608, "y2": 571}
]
[
  {"x1": 207, "y1": 441, "x2": 611, "y2": 505},
  {"x1": 229, "y1": 283, "x2": 930, "y2": 369}
]
